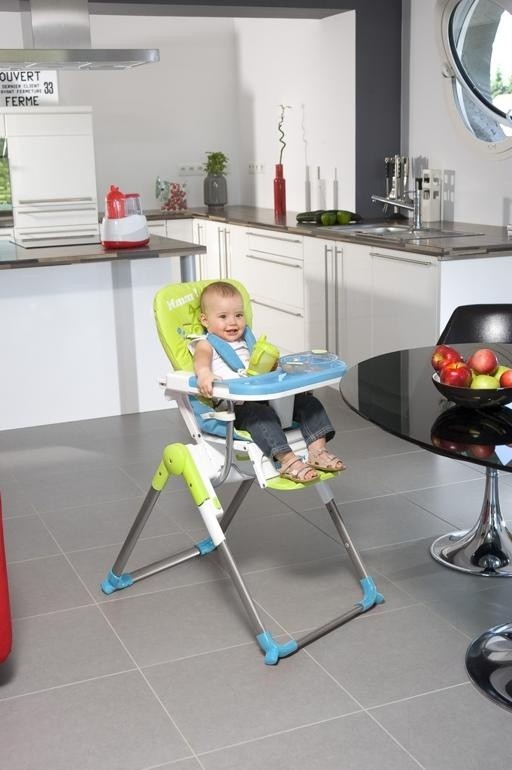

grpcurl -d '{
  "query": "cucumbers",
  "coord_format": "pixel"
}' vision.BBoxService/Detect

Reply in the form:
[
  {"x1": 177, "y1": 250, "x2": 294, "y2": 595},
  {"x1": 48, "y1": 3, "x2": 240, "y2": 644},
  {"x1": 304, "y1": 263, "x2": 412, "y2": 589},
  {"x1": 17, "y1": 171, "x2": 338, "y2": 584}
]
[
  {"x1": 315, "y1": 211, "x2": 361, "y2": 223},
  {"x1": 297, "y1": 206, "x2": 321, "y2": 223}
]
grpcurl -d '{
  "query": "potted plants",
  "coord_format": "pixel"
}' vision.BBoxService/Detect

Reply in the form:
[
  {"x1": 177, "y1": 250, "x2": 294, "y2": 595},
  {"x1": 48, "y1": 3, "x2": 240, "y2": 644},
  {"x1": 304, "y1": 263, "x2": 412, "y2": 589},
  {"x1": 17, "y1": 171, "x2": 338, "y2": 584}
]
[
  {"x1": 201, "y1": 151, "x2": 231, "y2": 209},
  {"x1": 270, "y1": 103, "x2": 294, "y2": 225}
]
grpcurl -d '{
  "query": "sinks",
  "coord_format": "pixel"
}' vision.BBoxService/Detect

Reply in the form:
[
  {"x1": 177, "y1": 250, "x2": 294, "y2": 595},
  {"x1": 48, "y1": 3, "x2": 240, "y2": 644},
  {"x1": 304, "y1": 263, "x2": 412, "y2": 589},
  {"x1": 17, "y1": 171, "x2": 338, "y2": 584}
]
[{"x1": 316, "y1": 221, "x2": 411, "y2": 237}]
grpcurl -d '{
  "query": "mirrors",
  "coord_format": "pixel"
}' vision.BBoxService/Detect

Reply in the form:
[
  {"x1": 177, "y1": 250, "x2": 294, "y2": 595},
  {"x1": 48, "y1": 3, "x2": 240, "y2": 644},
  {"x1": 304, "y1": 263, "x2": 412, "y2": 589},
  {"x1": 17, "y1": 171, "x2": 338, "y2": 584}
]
[{"x1": 439, "y1": 0, "x2": 512, "y2": 142}]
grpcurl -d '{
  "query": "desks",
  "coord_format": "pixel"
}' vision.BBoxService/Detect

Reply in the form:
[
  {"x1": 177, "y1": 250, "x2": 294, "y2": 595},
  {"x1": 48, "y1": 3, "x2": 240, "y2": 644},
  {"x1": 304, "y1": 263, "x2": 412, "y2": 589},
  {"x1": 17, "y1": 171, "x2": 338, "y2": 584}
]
[
  {"x1": 184, "y1": 347, "x2": 348, "y2": 422},
  {"x1": 1, "y1": 235, "x2": 210, "y2": 435}
]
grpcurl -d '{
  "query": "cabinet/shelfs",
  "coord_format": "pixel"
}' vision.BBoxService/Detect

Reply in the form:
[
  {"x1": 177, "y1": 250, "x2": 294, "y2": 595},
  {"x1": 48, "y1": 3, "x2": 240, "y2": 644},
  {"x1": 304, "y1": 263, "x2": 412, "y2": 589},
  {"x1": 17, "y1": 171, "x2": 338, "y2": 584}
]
[
  {"x1": 194, "y1": 219, "x2": 246, "y2": 297},
  {"x1": 304, "y1": 239, "x2": 368, "y2": 394},
  {"x1": 154, "y1": 215, "x2": 197, "y2": 249},
  {"x1": 368, "y1": 246, "x2": 512, "y2": 359},
  {"x1": 3, "y1": 112, "x2": 101, "y2": 240},
  {"x1": 246, "y1": 223, "x2": 309, "y2": 365}
]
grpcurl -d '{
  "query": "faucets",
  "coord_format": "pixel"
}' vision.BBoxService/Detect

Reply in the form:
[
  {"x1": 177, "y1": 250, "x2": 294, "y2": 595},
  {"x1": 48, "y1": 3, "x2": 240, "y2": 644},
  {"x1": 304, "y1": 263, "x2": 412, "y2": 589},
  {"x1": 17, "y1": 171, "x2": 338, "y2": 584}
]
[{"x1": 370, "y1": 193, "x2": 422, "y2": 230}]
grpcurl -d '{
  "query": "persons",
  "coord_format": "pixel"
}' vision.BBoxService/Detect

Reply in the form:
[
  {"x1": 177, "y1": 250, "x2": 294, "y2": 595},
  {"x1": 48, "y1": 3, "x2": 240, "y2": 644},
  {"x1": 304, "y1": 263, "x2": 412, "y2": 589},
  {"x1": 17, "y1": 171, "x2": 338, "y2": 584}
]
[{"x1": 194, "y1": 281, "x2": 347, "y2": 485}]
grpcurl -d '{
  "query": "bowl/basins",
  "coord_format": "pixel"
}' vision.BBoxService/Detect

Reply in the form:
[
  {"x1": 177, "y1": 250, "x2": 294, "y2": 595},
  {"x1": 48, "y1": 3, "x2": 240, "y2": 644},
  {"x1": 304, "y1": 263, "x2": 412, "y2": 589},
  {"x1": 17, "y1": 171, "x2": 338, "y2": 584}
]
[
  {"x1": 432, "y1": 365, "x2": 511, "y2": 409},
  {"x1": 429, "y1": 404, "x2": 512, "y2": 445}
]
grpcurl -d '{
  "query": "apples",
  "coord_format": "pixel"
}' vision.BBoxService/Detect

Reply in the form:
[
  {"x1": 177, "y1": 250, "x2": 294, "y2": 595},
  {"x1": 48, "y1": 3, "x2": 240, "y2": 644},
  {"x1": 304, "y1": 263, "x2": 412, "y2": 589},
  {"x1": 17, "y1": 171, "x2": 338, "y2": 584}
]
[
  {"x1": 441, "y1": 440, "x2": 464, "y2": 451},
  {"x1": 468, "y1": 444, "x2": 491, "y2": 458},
  {"x1": 431, "y1": 344, "x2": 512, "y2": 389},
  {"x1": 431, "y1": 436, "x2": 441, "y2": 447}
]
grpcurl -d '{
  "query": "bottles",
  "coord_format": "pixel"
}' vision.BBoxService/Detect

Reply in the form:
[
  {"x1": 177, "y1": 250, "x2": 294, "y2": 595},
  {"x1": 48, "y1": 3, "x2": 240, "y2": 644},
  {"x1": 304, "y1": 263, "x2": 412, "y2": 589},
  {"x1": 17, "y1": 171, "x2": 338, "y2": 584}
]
[{"x1": 247, "y1": 335, "x2": 280, "y2": 377}]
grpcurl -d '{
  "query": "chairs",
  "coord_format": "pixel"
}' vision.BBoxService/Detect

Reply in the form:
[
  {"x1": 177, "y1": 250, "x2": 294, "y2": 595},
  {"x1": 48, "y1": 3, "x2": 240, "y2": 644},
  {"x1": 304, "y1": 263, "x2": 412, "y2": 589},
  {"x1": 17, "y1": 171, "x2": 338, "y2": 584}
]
[
  {"x1": 417, "y1": 299, "x2": 512, "y2": 586},
  {"x1": 150, "y1": 277, "x2": 350, "y2": 502}
]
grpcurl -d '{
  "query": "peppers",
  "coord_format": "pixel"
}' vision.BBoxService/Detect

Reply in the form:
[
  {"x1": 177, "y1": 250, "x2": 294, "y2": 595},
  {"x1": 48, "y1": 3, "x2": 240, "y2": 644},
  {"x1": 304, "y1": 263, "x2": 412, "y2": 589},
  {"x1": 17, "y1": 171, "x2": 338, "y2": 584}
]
[
  {"x1": 321, "y1": 213, "x2": 336, "y2": 226},
  {"x1": 336, "y1": 211, "x2": 352, "y2": 224}
]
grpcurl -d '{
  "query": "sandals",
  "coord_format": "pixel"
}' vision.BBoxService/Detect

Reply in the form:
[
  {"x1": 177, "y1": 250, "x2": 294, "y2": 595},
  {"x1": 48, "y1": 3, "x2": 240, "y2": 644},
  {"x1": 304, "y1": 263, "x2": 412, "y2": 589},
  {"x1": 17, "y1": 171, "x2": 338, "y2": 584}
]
[{"x1": 276, "y1": 447, "x2": 348, "y2": 483}]
